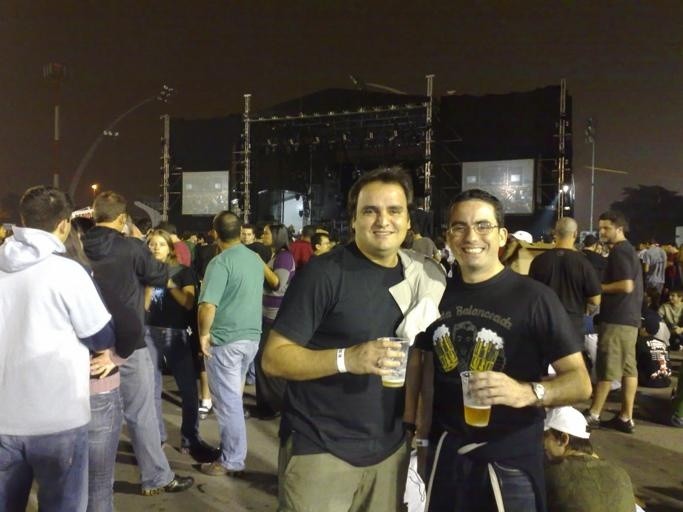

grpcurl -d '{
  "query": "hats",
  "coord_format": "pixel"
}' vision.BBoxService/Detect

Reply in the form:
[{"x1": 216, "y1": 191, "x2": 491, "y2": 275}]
[{"x1": 510, "y1": 230, "x2": 533, "y2": 243}]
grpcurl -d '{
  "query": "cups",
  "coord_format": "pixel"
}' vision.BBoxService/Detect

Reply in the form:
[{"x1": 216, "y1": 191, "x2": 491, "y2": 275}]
[
  {"x1": 468, "y1": 337, "x2": 501, "y2": 374},
  {"x1": 459, "y1": 371, "x2": 492, "y2": 428},
  {"x1": 377, "y1": 336, "x2": 410, "y2": 389},
  {"x1": 432, "y1": 334, "x2": 457, "y2": 374}
]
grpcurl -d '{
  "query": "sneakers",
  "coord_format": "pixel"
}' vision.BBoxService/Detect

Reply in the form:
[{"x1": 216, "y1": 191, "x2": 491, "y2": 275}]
[
  {"x1": 201, "y1": 460, "x2": 244, "y2": 476},
  {"x1": 181, "y1": 437, "x2": 212, "y2": 462},
  {"x1": 199, "y1": 403, "x2": 213, "y2": 414},
  {"x1": 544, "y1": 406, "x2": 591, "y2": 440},
  {"x1": 582, "y1": 408, "x2": 600, "y2": 429},
  {"x1": 141, "y1": 474, "x2": 193, "y2": 496},
  {"x1": 601, "y1": 417, "x2": 635, "y2": 433}
]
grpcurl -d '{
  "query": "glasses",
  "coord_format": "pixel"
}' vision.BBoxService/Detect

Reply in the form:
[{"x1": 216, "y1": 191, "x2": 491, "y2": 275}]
[{"x1": 448, "y1": 223, "x2": 500, "y2": 235}]
